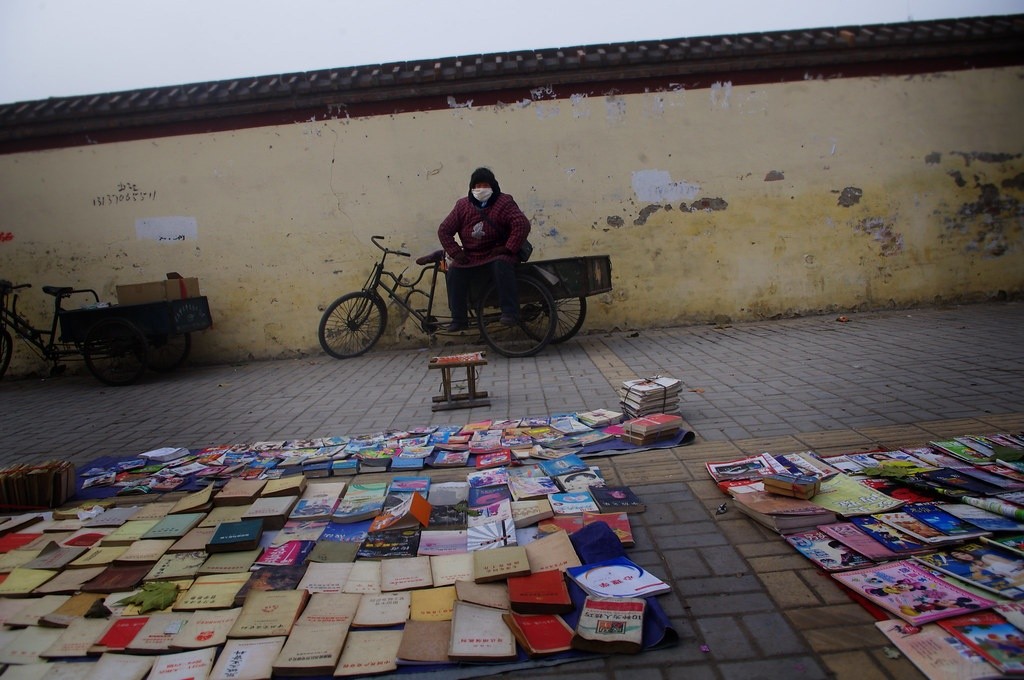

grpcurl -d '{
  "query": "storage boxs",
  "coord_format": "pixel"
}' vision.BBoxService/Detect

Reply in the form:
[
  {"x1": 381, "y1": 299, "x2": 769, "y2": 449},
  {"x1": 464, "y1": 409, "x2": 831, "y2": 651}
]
[{"x1": 116, "y1": 273, "x2": 200, "y2": 306}]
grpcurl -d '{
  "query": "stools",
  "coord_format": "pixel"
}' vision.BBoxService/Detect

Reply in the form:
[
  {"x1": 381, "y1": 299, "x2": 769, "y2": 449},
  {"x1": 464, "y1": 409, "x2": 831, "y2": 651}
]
[{"x1": 428, "y1": 348, "x2": 490, "y2": 412}]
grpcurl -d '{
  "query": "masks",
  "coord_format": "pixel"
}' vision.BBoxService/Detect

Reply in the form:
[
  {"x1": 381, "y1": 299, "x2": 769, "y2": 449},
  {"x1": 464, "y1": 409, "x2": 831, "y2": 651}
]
[{"x1": 472, "y1": 188, "x2": 493, "y2": 201}]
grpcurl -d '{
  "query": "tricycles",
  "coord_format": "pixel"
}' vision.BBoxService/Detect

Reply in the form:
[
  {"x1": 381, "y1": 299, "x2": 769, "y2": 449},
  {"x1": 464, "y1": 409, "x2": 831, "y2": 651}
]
[
  {"x1": 317, "y1": 235, "x2": 613, "y2": 357},
  {"x1": 0, "y1": 279, "x2": 213, "y2": 387}
]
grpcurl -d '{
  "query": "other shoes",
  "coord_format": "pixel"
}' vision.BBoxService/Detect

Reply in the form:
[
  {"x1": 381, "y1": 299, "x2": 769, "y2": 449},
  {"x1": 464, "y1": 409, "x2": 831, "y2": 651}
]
[
  {"x1": 500, "y1": 313, "x2": 520, "y2": 324},
  {"x1": 447, "y1": 318, "x2": 469, "y2": 333}
]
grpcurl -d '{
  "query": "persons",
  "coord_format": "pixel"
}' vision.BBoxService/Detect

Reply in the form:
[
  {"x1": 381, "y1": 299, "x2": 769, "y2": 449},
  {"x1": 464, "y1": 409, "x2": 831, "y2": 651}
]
[{"x1": 438, "y1": 167, "x2": 531, "y2": 333}]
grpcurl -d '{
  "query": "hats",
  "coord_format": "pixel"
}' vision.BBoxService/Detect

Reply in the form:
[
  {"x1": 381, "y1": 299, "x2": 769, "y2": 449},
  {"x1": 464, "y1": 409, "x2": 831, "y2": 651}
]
[{"x1": 470, "y1": 167, "x2": 496, "y2": 189}]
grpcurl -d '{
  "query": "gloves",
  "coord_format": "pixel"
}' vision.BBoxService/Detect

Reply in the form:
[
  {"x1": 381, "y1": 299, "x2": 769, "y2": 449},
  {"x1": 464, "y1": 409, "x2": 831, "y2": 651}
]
[
  {"x1": 454, "y1": 251, "x2": 468, "y2": 265},
  {"x1": 504, "y1": 248, "x2": 513, "y2": 257}
]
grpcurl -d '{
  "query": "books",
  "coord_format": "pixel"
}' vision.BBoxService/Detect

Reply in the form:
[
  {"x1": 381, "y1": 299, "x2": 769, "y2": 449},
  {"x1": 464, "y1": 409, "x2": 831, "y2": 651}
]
[
  {"x1": 0, "y1": 375, "x2": 684, "y2": 680},
  {"x1": 705, "y1": 429, "x2": 1024, "y2": 680}
]
